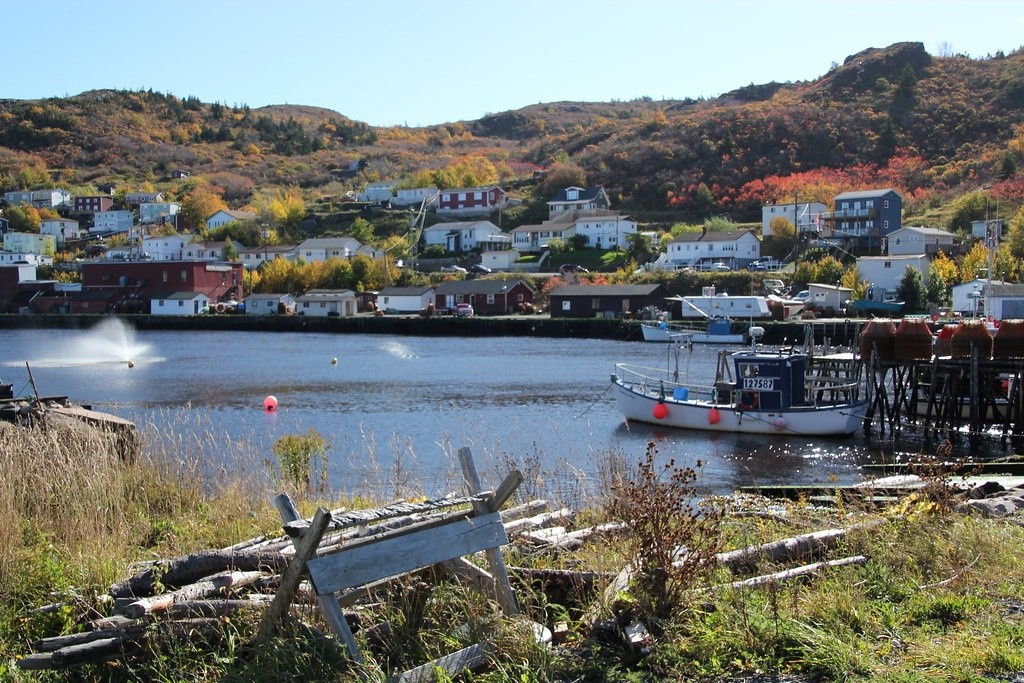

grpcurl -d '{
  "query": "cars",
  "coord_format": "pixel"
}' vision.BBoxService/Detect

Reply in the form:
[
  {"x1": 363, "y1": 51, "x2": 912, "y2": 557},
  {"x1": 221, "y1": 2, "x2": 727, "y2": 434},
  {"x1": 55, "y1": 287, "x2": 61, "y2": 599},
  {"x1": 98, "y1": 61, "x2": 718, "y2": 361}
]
[
  {"x1": 440, "y1": 264, "x2": 466, "y2": 273},
  {"x1": 559, "y1": 263, "x2": 590, "y2": 276},
  {"x1": 468, "y1": 263, "x2": 492, "y2": 275},
  {"x1": 451, "y1": 304, "x2": 475, "y2": 317},
  {"x1": 710, "y1": 262, "x2": 730, "y2": 272}
]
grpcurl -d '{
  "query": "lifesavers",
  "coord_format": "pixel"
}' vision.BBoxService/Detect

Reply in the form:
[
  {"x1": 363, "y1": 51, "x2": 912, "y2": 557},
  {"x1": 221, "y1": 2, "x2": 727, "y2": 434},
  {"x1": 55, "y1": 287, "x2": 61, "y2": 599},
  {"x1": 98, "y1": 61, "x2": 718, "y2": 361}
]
[{"x1": 214, "y1": 301, "x2": 226, "y2": 314}]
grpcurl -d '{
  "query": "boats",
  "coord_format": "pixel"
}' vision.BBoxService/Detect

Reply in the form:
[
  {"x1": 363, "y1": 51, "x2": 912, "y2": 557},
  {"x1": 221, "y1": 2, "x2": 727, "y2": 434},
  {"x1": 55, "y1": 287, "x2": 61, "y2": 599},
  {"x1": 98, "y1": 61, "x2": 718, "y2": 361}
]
[{"x1": 608, "y1": 191, "x2": 1023, "y2": 437}]
